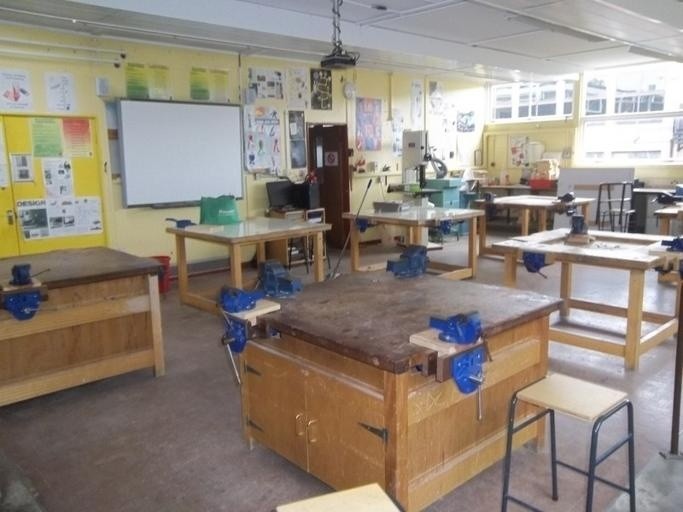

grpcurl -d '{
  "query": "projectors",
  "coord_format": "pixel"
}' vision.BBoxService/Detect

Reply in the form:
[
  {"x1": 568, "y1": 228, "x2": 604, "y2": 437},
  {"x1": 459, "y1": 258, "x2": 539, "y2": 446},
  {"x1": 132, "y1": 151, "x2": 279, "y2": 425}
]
[{"x1": 321, "y1": 47, "x2": 361, "y2": 71}]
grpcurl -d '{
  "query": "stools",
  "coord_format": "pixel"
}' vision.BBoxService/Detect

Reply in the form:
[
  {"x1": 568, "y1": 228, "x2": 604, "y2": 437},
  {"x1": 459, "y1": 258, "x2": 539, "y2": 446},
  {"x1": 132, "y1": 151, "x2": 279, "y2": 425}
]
[
  {"x1": 272, "y1": 482, "x2": 405, "y2": 512},
  {"x1": 501, "y1": 374, "x2": 636, "y2": 511},
  {"x1": 597, "y1": 180, "x2": 633, "y2": 231},
  {"x1": 289, "y1": 208, "x2": 331, "y2": 274}
]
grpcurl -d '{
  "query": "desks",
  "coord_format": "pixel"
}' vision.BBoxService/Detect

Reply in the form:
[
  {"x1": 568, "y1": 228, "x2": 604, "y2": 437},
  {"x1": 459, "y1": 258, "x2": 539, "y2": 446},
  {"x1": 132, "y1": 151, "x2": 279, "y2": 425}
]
[
  {"x1": 474, "y1": 195, "x2": 596, "y2": 259},
  {"x1": 267, "y1": 209, "x2": 327, "y2": 265},
  {"x1": 654, "y1": 202, "x2": 683, "y2": 285},
  {"x1": 480, "y1": 184, "x2": 557, "y2": 224},
  {"x1": 166, "y1": 215, "x2": 333, "y2": 317},
  {"x1": 0, "y1": 247, "x2": 169, "y2": 407},
  {"x1": 342, "y1": 207, "x2": 485, "y2": 281},
  {"x1": 240, "y1": 267, "x2": 565, "y2": 512},
  {"x1": 491, "y1": 224, "x2": 683, "y2": 371}
]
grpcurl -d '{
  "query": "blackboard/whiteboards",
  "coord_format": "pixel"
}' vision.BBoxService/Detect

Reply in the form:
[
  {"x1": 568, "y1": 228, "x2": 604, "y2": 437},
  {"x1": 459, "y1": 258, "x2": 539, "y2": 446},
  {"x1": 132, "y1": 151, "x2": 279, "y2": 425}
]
[{"x1": 115, "y1": 96, "x2": 245, "y2": 209}]
